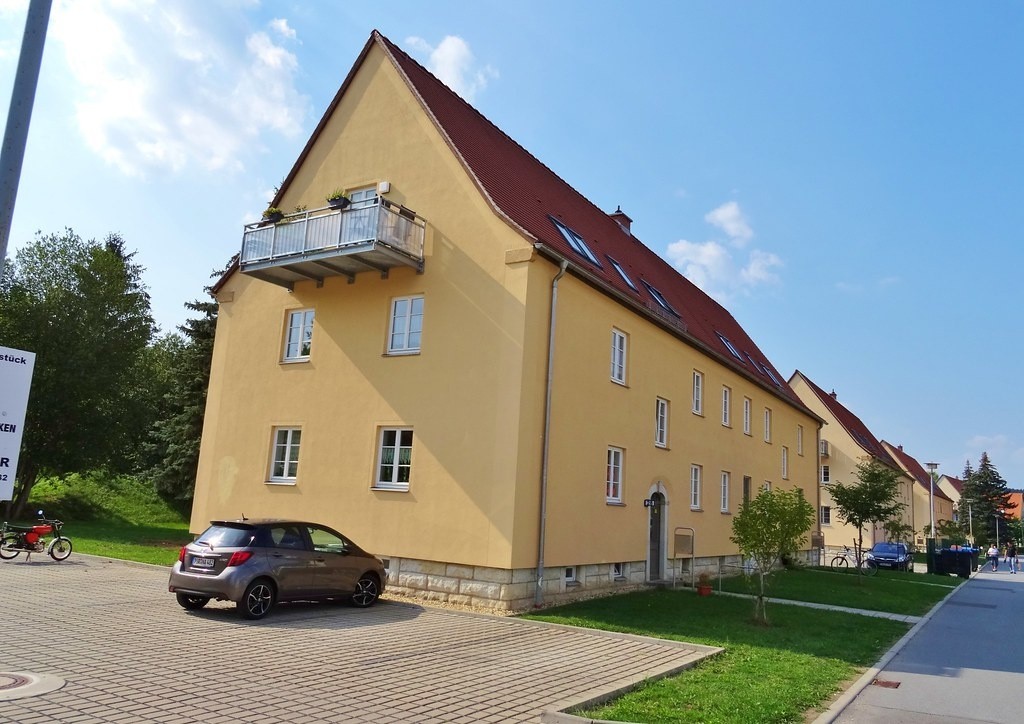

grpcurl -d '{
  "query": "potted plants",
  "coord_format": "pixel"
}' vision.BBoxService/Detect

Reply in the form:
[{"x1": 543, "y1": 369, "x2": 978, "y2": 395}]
[
  {"x1": 697, "y1": 574, "x2": 712, "y2": 598},
  {"x1": 322, "y1": 186, "x2": 353, "y2": 210},
  {"x1": 261, "y1": 207, "x2": 284, "y2": 222}
]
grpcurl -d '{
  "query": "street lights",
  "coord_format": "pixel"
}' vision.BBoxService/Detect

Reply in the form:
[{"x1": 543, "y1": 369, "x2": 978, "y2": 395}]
[
  {"x1": 924, "y1": 462, "x2": 940, "y2": 539},
  {"x1": 994, "y1": 514, "x2": 1000, "y2": 551},
  {"x1": 965, "y1": 496, "x2": 975, "y2": 549}
]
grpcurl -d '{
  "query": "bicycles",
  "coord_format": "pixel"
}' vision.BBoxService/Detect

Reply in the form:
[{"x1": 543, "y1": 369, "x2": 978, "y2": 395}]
[{"x1": 831, "y1": 544, "x2": 879, "y2": 576}]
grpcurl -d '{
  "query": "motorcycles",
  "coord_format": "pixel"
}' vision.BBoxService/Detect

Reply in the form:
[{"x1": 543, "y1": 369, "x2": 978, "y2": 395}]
[{"x1": 0, "y1": 510, "x2": 72, "y2": 564}]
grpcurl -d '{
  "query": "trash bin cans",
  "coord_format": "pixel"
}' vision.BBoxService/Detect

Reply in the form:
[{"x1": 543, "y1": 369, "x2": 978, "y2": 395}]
[{"x1": 965, "y1": 549, "x2": 979, "y2": 569}]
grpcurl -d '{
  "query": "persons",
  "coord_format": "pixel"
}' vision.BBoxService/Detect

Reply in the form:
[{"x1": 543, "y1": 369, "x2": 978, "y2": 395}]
[
  {"x1": 1003, "y1": 541, "x2": 1021, "y2": 574},
  {"x1": 988, "y1": 543, "x2": 998, "y2": 572}
]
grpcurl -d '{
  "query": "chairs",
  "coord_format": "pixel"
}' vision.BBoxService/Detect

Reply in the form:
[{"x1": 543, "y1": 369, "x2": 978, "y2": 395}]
[
  {"x1": 279, "y1": 530, "x2": 303, "y2": 548},
  {"x1": 252, "y1": 527, "x2": 275, "y2": 546}
]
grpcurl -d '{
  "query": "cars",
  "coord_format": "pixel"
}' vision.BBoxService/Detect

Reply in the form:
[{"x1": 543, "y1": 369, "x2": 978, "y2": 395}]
[
  {"x1": 167, "y1": 517, "x2": 390, "y2": 621},
  {"x1": 866, "y1": 541, "x2": 914, "y2": 574}
]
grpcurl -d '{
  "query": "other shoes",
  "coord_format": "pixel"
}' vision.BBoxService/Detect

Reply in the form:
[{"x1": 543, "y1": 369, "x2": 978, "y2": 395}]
[
  {"x1": 1009, "y1": 571, "x2": 1013, "y2": 575},
  {"x1": 1014, "y1": 571, "x2": 1016, "y2": 574},
  {"x1": 994, "y1": 569, "x2": 997, "y2": 571},
  {"x1": 992, "y1": 569, "x2": 994, "y2": 572}
]
